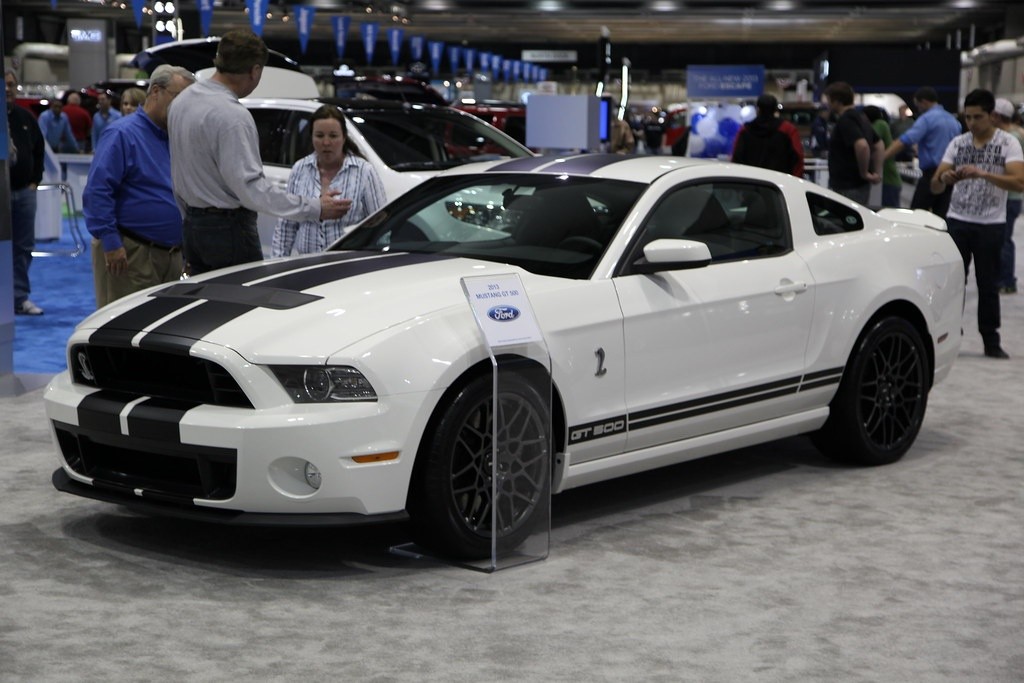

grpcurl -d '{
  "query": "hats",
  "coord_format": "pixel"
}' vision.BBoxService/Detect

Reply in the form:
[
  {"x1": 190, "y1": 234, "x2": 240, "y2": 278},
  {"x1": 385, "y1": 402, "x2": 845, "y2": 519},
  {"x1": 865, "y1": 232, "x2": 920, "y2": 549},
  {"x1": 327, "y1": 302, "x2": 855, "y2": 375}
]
[
  {"x1": 992, "y1": 98, "x2": 1014, "y2": 117},
  {"x1": 818, "y1": 103, "x2": 828, "y2": 110}
]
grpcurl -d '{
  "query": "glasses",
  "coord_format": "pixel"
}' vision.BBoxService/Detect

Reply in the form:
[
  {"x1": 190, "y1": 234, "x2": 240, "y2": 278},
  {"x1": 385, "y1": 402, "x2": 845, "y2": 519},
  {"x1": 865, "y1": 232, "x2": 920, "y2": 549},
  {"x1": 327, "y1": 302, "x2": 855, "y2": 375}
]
[{"x1": 156, "y1": 83, "x2": 180, "y2": 98}]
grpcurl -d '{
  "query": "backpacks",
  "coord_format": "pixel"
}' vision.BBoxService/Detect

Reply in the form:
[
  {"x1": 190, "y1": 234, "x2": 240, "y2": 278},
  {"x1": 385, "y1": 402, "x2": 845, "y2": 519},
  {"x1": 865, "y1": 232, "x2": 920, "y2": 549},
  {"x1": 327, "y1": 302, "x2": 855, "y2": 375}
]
[{"x1": 732, "y1": 116, "x2": 784, "y2": 171}]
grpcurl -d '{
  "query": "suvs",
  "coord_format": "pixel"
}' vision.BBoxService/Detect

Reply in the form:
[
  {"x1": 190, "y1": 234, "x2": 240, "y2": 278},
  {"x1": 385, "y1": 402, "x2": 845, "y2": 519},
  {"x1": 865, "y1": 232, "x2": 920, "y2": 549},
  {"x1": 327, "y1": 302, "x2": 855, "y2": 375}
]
[{"x1": 130, "y1": 34, "x2": 610, "y2": 250}]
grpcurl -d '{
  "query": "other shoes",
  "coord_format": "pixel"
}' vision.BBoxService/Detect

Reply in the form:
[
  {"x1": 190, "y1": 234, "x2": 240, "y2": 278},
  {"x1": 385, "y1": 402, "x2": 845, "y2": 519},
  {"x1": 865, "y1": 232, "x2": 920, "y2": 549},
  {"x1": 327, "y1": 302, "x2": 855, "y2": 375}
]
[
  {"x1": 15, "y1": 301, "x2": 44, "y2": 314},
  {"x1": 985, "y1": 346, "x2": 1009, "y2": 358},
  {"x1": 998, "y1": 286, "x2": 1017, "y2": 294}
]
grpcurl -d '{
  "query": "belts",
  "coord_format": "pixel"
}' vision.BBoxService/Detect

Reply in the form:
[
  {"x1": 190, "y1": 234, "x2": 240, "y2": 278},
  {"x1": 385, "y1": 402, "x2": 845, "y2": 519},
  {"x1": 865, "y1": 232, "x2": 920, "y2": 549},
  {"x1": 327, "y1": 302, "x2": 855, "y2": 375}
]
[{"x1": 116, "y1": 225, "x2": 183, "y2": 254}]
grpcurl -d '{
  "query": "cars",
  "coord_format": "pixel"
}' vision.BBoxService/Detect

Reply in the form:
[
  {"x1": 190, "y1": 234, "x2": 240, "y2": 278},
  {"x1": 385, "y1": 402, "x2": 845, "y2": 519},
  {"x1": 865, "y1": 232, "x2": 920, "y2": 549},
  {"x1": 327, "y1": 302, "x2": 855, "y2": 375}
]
[
  {"x1": 777, "y1": 102, "x2": 831, "y2": 158},
  {"x1": 17, "y1": 65, "x2": 525, "y2": 148},
  {"x1": 626, "y1": 102, "x2": 688, "y2": 144},
  {"x1": 40, "y1": 153, "x2": 967, "y2": 559}
]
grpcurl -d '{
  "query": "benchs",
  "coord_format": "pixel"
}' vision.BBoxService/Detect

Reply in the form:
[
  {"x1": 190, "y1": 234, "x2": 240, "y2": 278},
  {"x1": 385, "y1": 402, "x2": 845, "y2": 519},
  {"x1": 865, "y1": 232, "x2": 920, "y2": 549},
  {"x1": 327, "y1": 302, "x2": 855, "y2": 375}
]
[{"x1": 659, "y1": 192, "x2": 783, "y2": 252}]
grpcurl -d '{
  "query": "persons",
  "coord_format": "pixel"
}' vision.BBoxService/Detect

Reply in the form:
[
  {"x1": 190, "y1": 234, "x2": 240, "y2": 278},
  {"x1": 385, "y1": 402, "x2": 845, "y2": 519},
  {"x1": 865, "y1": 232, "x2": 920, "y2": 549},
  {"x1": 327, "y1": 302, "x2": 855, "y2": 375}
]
[
  {"x1": 37, "y1": 98, "x2": 82, "y2": 154},
  {"x1": 930, "y1": 89, "x2": 1024, "y2": 359},
  {"x1": 272, "y1": 105, "x2": 387, "y2": 258},
  {"x1": 167, "y1": 29, "x2": 353, "y2": 274},
  {"x1": 627, "y1": 107, "x2": 685, "y2": 156},
  {"x1": 610, "y1": 102, "x2": 635, "y2": 155},
  {"x1": 4, "y1": 67, "x2": 44, "y2": 316},
  {"x1": 991, "y1": 99, "x2": 1024, "y2": 294},
  {"x1": 91, "y1": 91, "x2": 122, "y2": 154},
  {"x1": 733, "y1": 94, "x2": 804, "y2": 207},
  {"x1": 863, "y1": 105, "x2": 901, "y2": 210},
  {"x1": 121, "y1": 87, "x2": 146, "y2": 116},
  {"x1": 81, "y1": 63, "x2": 198, "y2": 309},
  {"x1": 825, "y1": 81, "x2": 884, "y2": 216},
  {"x1": 1011, "y1": 105, "x2": 1024, "y2": 127},
  {"x1": 885, "y1": 86, "x2": 963, "y2": 217},
  {"x1": 891, "y1": 107, "x2": 915, "y2": 162},
  {"x1": 61, "y1": 93, "x2": 91, "y2": 154},
  {"x1": 809, "y1": 105, "x2": 830, "y2": 156}
]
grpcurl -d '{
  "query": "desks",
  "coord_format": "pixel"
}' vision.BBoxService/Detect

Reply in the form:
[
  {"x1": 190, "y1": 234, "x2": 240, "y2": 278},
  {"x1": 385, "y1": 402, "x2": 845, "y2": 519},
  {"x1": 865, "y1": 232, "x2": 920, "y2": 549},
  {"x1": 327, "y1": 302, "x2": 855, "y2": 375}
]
[
  {"x1": 56, "y1": 152, "x2": 95, "y2": 213},
  {"x1": 805, "y1": 158, "x2": 829, "y2": 188},
  {"x1": 894, "y1": 159, "x2": 925, "y2": 208}
]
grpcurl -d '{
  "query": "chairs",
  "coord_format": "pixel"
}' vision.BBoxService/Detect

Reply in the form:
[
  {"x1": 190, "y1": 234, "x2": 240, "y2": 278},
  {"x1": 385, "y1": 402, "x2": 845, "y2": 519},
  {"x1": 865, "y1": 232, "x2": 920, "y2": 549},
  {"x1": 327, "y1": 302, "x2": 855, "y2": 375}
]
[
  {"x1": 31, "y1": 182, "x2": 87, "y2": 257},
  {"x1": 513, "y1": 182, "x2": 607, "y2": 256}
]
